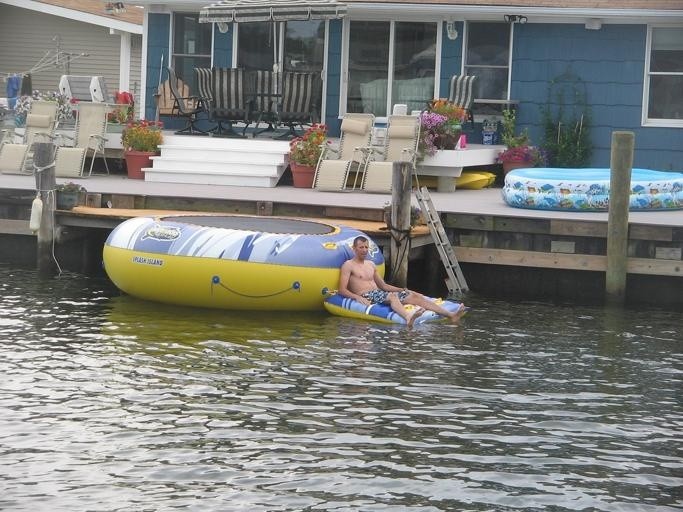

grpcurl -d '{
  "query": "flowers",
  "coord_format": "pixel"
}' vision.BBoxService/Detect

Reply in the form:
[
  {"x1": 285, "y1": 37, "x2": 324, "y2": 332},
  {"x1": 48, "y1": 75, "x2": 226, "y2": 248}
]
[
  {"x1": 289, "y1": 123, "x2": 332, "y2": 168},
  {"x1": 418, "y1": 99, "x2": 469, "y2": 161},
  {"x1": 120, "y1": 120, "x2": 164, "y2": 151},
  {"x1": 495, "y1": 110, "x2": 547, "y2": 168}
]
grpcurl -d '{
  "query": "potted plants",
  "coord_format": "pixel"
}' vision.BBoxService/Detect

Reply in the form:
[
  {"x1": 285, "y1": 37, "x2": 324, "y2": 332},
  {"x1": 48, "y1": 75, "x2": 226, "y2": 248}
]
[
  {"x1": 55, "y1": 182, "x2": 81, "y2": 208},
  {"x1": 443, "y1": 123, "x2": 463, "y2": 150}
]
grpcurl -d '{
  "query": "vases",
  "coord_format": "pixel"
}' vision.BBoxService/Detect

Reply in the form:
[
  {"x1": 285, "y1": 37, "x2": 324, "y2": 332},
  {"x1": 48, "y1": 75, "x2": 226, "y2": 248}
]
[
  {"x1": 124, "y1": 152, "x2": 157, "y2": 179},
  {"x1": 503, "y1": 160, "x2": 537, "y2": 177},
  {"x1": 288, "y1": 163, "x2": 316, "y2": 189}
]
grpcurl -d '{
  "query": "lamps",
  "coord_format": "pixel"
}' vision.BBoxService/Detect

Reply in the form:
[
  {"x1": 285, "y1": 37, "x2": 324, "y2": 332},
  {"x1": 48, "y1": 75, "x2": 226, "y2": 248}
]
[
  {"x1": 504, "y1": 15, "x2": 527, "y2": 24},
  {"x1": 585, "y1": 19, "x2": 602, "y2": 30},
  {"x1": 106, "y1": 3, "x2": 127, "y2": 13}
]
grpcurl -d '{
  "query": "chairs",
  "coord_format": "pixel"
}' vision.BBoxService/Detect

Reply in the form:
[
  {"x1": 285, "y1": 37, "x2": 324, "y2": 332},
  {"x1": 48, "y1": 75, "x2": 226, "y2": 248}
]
[
  {"x1": 153, "y1": 65, "x2": 320, "y2": 140},
  {"x1": 421, "y1": 75, "x2": 478, "y2": 129},
  {"x1": 0, "y1": 99, "x2": 59, "y2": 176},
  {"x1": 312, "y1": 113, "x2": 376, "y2": 193},
  {"x1": 360, "y1": 114, "x2": 421, "y2": 195},
  {"x1": 54, "y1": 102, "x2": 110, "y2": 180}
]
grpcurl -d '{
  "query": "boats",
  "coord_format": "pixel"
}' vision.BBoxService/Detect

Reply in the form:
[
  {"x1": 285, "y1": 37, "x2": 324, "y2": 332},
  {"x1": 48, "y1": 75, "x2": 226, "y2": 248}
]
[
  {"x1": 346, "y1": 170, "x2": 498, "y2": 190},
  {"x1": 319, "y1": 289, "x2": 473, "y2": 328},
  {"x1": 500, "y1": 164, "x2": 683, "y2": 213},
  {"x1": 101, "y1": 212, "x2": 388, "y2": 314}
]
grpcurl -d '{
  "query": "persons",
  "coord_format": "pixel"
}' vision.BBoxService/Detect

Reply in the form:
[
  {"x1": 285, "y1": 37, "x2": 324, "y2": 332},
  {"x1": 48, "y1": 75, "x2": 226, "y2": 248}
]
[{"x1": 339, "y1": 236, "x2": 466, "y2": 326}]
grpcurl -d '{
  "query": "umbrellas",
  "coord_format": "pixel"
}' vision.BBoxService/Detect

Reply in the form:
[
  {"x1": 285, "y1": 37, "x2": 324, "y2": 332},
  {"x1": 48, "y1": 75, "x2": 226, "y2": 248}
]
[{"x1": 198, "y1": 0, "x2": 349, "y2": 130}]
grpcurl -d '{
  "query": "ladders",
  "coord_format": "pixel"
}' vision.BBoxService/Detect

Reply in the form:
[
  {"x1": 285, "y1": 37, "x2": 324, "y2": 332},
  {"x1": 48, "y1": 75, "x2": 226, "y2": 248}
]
[{"x1": 412, "y1": 185, "x2": 470, "y2": 294}]
